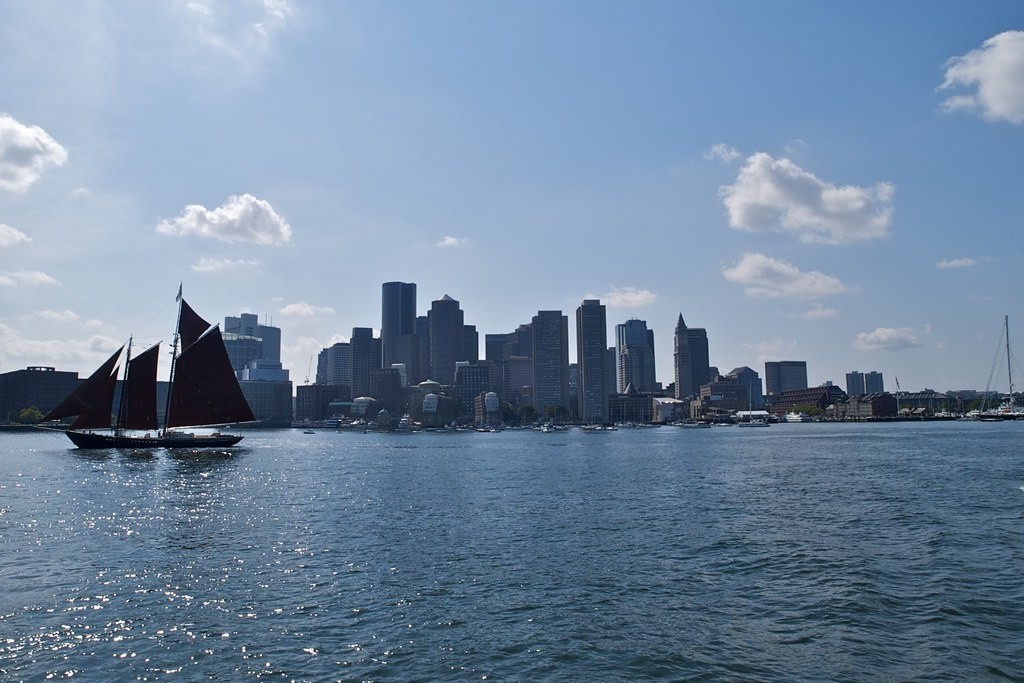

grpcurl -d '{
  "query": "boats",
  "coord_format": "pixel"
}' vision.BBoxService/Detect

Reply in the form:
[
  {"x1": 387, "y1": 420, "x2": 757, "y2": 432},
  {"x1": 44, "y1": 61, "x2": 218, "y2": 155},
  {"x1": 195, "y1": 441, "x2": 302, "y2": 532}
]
[
  {"x1": 379, "y1": 429, "x2": 392, "y2": 433},
  {"x1": 715, "y1": 422, "x2": 733, "y2": 426},
  {"x1": 0, "y1": 420, "x2": 75, "y2": 431},
  {"x1": 366, "y1": 428, "x2": 373, "y2": 432},
  {"x1": 335, "y1": 429, "x2": 343, "y2": 434},
  {"x1": 394, "y1": 414, "x2": 414, "y2": 433},
  {"x1": 785, "y1": 410, "x2": 812, "y2": 423},
  {"x1": 683, "y1": 421, "x2": 712, "y2": 429},
  {"x1": 303, "y1": 429, "x2": 315, "y2": 434},
  {"x1": 738, "y1": 411, "x2": 772, "y2": 427},
  {"x1": 413, "y1": 421, "x2": 661, "y2": 433}
]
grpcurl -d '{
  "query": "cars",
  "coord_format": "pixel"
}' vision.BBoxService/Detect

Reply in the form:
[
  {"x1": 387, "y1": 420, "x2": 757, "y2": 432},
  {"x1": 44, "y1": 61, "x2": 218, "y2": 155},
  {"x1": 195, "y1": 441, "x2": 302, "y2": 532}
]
[
  {"x1": 898, "y1": 408, "x2": 980, "y2": 418},
  {"x1": 367, "y1": 421, "x2": 378, "y2": 427}
]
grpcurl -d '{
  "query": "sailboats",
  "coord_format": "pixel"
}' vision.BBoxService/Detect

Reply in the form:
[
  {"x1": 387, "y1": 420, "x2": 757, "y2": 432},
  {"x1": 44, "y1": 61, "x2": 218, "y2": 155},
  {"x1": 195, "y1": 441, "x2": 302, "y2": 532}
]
[
  {"x1": 973, "y1": 314, "x2": 1024, "y2": 421},
  {"x1": 35, "y1": 281, "x2": 257, "y2": 448}
]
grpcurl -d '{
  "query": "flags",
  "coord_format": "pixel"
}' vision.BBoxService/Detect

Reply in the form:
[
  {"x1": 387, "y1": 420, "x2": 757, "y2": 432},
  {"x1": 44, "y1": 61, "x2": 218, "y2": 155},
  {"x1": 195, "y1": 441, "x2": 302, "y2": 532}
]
[
  {"x1": 896, "y1": 377, "x2": 900, "y2": 389},
  {"x1": 176, "y1": 284, "x2": 182, "y2": 300}
]
